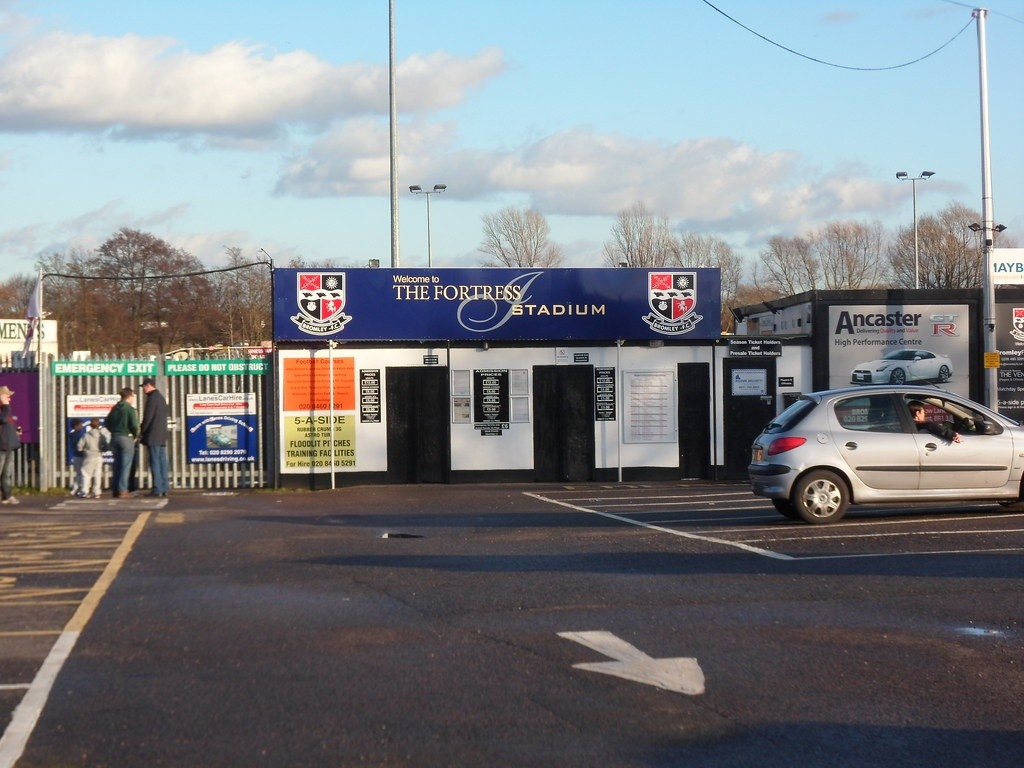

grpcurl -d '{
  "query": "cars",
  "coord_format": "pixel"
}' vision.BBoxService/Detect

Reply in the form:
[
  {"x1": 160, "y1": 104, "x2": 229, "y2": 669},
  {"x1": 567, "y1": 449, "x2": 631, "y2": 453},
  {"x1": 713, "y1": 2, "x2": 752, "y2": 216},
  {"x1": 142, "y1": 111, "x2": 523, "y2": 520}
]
[
  {"x1": 747, "y1": 384, "x2": 1023, "y2": 525},
  {"x1": 211, "y1": 433, "x2": 232, "y2": 447}
]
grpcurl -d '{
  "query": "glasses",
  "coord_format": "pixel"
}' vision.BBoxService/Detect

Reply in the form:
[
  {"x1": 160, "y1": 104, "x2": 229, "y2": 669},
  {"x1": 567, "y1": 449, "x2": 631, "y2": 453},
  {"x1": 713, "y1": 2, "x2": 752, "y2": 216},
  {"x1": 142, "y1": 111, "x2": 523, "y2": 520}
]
[{"x1": 922, "y1": 411, "x2": 928, "y2": 416}]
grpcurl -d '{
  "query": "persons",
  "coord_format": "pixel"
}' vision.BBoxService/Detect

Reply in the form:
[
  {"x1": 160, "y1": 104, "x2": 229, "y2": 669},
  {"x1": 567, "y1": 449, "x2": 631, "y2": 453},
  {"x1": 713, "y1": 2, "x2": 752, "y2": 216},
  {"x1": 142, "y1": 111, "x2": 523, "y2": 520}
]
[
  {"x1": 0, "y1": 386, "x2": 22, "y2": 505},
  {"x1": 77, "y1": 418, "x2": 108, "y2": 499},
  {"x1": 138, "y1": 378, "x2": 169, "y2": 498},
  {"x1": 907, "y1": 401, "x2": 961, "y2": 443},
  {"x1": 104, "y1": 387, "x2": 138, "y2": 498}
]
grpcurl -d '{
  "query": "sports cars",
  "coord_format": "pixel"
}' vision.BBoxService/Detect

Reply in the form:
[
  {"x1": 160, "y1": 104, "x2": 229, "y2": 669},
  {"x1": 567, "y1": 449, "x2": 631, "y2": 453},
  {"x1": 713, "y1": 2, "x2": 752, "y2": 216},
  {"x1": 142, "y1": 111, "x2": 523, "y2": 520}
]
[{"x1": 850, "y1": 347, "x2": 954, "y2": 385}]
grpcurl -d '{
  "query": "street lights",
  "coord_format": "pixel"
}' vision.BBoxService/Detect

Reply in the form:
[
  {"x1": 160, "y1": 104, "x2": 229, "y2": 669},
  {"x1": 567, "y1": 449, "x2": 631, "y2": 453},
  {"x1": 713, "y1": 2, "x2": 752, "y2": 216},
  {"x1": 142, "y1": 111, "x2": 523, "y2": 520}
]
[
  {"x1": 410, "y1": 184, "x2": 447, "y2": 268},
  {"x1": 970, "y1": 222, "x2": 1007, "y2": 414},
  {"x1": 896, "y1": 171, "x2": 937, "y2": 289}
]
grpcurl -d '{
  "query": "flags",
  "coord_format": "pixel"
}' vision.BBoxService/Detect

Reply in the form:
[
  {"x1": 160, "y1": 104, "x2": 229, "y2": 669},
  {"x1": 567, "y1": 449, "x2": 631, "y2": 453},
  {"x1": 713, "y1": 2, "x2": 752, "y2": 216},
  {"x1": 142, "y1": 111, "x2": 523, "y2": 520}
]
[{"x1": 21, "y1": 270, "x2": 41, "y2": 359}]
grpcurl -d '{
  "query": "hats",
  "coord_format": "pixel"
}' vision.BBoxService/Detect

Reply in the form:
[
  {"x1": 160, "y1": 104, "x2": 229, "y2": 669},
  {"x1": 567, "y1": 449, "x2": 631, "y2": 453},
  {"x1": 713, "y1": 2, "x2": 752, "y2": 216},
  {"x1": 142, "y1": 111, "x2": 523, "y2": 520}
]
[
  {"x1": 138, "y1": 378, "x2": 155, "y2": 387},
  {"x1": 0, "y1": 386, "x2": 14, "y2": 396}
]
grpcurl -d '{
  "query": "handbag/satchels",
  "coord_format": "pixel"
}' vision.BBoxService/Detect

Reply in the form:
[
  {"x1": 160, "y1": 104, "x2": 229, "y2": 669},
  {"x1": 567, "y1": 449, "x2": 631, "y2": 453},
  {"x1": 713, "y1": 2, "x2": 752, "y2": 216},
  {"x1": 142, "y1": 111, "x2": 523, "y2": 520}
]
[{"x1": 99, "y1": 431, "x2": 109, "y2": 451}]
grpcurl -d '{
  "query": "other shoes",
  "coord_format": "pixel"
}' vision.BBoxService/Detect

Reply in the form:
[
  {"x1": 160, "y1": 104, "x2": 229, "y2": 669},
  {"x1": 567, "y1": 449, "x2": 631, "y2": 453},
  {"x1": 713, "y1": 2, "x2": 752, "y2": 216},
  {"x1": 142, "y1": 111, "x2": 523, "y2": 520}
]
[
  {"x1": 76, "y1": 490, "x2": 91, "y2": 498},
  {"x1": 1, "y1": 496, "x2": 20, "y2": 505},
  {"x1": 94, "y1": 495, "x2": 101, "y2": 499},
  {"x1": 163, "y1": 492, "x2": 168, "y2": 496},
  {"x1": 112, "y1": 491, "x2": 135, "y2": 498},
  {"x1": 144, "y1": 490, "x2": 162, "y2": 497}
]
